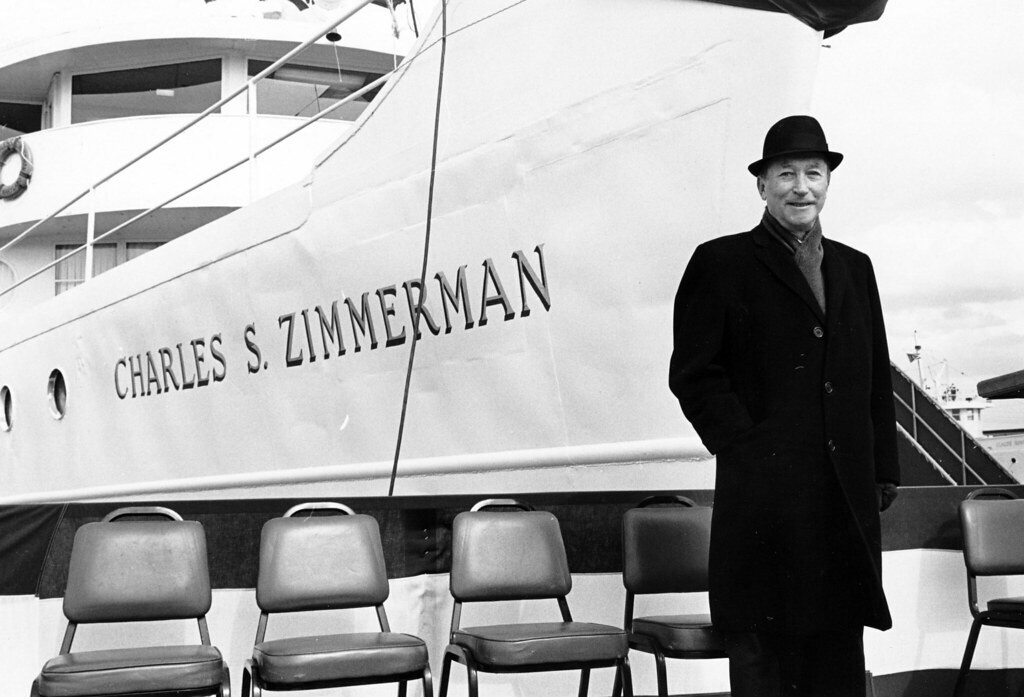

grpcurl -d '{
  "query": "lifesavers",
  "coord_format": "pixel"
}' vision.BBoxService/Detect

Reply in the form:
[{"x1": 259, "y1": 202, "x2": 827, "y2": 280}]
[{"x1": 0, "y1": 139, "x2": 33, "y2": 201}]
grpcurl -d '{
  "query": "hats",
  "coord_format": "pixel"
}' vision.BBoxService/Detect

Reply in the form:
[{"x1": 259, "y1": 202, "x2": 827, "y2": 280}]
[{"x1": 747, "y1": 115, "x2": 843, "y2": 177}]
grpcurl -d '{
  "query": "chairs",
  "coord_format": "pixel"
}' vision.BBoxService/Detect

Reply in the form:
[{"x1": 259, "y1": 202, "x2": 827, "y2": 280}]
[
  {"x1": 612, "y1": 495, "x2": 732, "y2": 697},
  {"x1": 438, "y1": 499, "x2": 633, "y2": 697},
  {"x1": 30, "y1": 506, "x2": 231, "y2": 697},
  {"x1": 241, "y1": 502, "x2": 434, "y2": 697},
  {"x1": 957, "y1": 487, "x2": 1024, "y2": 678}
]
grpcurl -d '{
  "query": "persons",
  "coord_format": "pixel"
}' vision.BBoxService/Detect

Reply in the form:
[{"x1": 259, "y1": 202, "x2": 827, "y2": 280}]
[{"x1": 668, "y1": 115, "x2": 900, "y2": 697}]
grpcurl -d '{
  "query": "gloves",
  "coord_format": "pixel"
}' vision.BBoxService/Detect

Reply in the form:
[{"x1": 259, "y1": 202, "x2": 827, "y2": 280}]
[{"x1": 879, "y1": 484, "x2": 898, "y2": 511}]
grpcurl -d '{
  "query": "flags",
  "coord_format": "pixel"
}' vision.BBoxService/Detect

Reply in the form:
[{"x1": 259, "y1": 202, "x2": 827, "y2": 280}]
[{"x1": 907, "y1": 353, "x2": 918, "y2": 362}]
